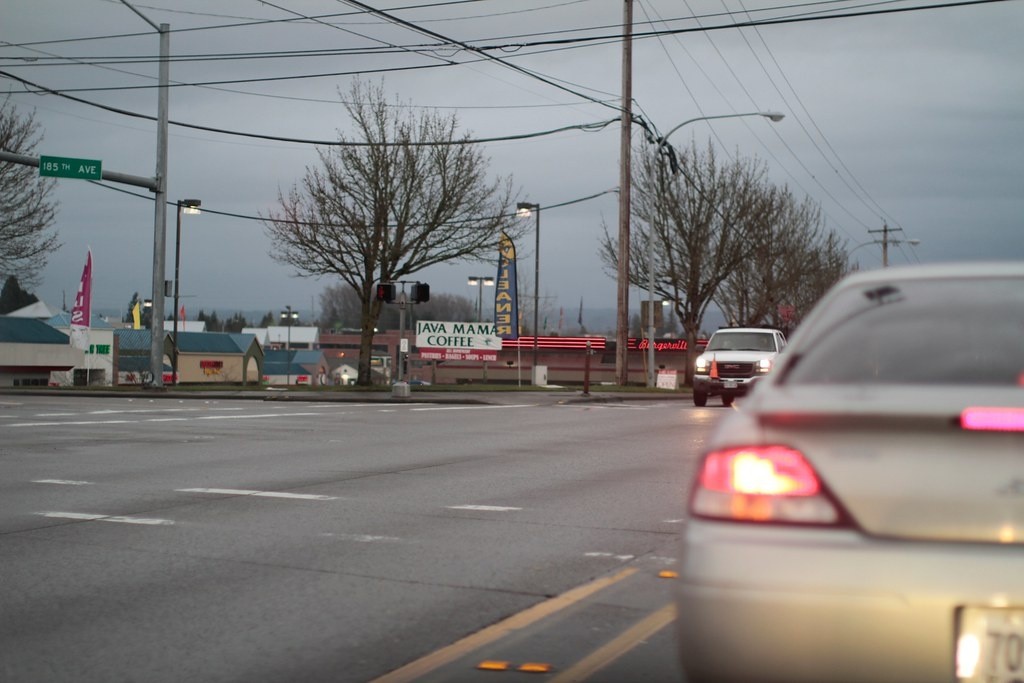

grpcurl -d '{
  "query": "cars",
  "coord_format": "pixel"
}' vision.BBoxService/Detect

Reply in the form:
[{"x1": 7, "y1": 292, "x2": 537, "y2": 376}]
[{"x1": 675, "y1": 262, "x2": 1023, "y2": 683}]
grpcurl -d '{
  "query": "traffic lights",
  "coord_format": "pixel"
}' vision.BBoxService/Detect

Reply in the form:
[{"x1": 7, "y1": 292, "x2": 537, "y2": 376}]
[
  {"x1": 376, "y1": 283, "x2": 397, "y2": 302},
  {"x1": 411, "y1": 284, "x2": 431, "y2": 302}
]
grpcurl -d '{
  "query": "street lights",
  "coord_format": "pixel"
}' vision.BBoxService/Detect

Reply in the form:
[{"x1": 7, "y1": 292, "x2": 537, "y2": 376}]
[
  {"x1": 645, "y1": 111, "x2": 787, "y2": 387},
  {"x1": 839, "y1": 237, "x2": 920, "y2": 276},
  {"x1": 468, "y1": 275, "x2": 495, "y2": 323},
  {"x1": 280, "y1": 305, "x2": 299, "y2": 386},
  {"x1": 516, "y1": 202, "x2": 541, "y2": 387},
  {"x1": 172, "y1": 199, "x2": 202, "y2": 387}
]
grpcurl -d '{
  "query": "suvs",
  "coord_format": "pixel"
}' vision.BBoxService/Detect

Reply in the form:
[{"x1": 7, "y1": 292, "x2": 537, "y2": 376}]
[{"x1": 692, "y1": 324, "x2": 789, "y2": 407}]
